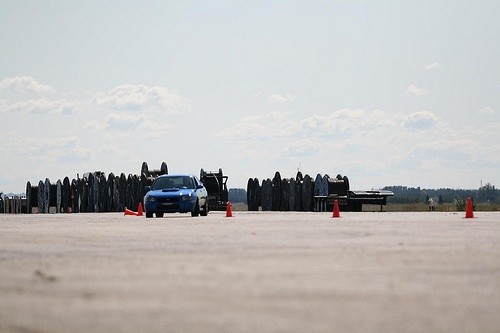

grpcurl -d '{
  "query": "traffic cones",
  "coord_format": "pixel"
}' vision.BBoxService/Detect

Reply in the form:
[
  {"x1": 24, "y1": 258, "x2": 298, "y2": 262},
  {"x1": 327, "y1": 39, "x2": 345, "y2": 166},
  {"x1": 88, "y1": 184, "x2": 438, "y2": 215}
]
[
  {"x1": 124, "y1": 207, "x2": 137, "y2": 216},
  {"x1": 136, "y1": 202, "x2": 144, "y2": 217},
  {"x1": 462, "y1": 195, "x2": 478, "y2": 219},
  {"x1": 330, "y1": 199, "x2": 342, "y2": 218},
  {"x1": 225, "y1": 201, "x2": 234, "y2": 217}
]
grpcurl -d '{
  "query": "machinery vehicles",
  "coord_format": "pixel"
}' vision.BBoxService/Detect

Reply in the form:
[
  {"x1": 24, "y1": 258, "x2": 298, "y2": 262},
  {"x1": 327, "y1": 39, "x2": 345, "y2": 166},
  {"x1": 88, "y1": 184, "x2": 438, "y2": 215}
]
[{"x1": 199, "y1": 169, "x2": 229, "y2": 211}]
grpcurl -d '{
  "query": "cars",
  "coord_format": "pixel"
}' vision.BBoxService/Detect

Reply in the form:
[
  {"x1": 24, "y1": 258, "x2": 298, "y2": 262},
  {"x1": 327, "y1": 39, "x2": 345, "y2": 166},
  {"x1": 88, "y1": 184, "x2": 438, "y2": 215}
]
[{"x1": 143, "y1": 174, "x2": 209, "y2": 217}]
